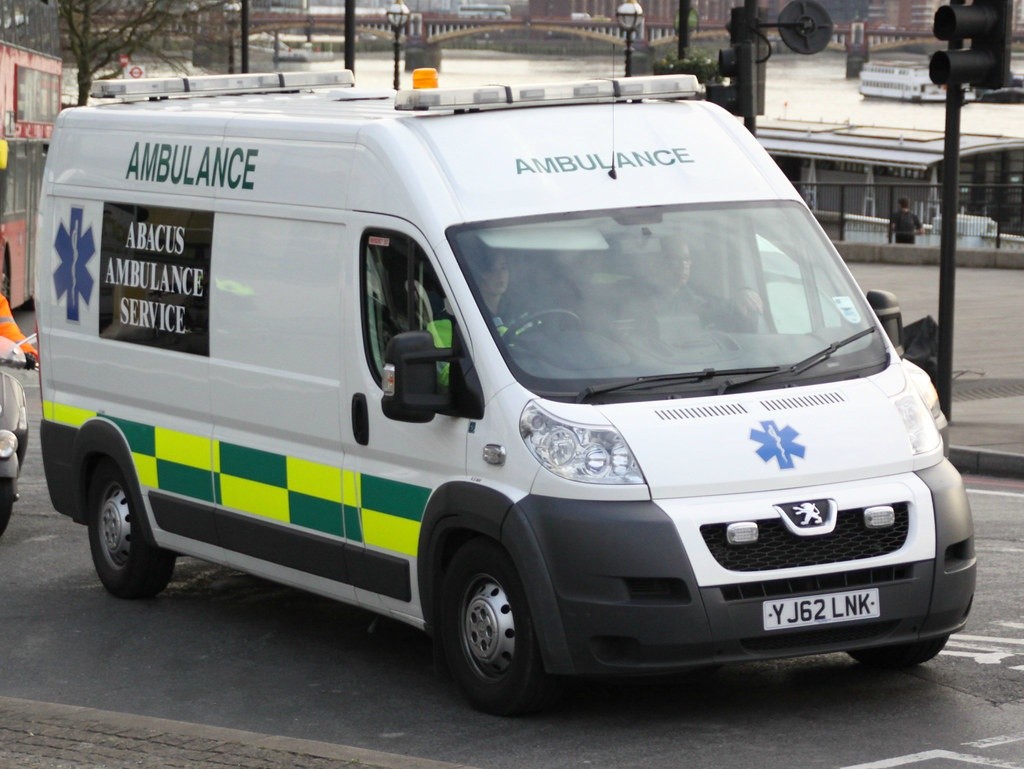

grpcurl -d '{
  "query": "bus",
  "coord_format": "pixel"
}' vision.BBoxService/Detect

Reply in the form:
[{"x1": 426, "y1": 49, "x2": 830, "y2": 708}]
[
  {"x1": 458, "y1": 4, "x2": 512, "y2": 23},
  {"x1": 0, "y1": 1, "x2": 64, "y2": 311}
]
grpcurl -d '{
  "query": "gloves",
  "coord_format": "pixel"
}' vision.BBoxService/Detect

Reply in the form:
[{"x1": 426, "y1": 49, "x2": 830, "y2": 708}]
[{"x1": 24, "y1": 352, "x2": 36, "y2": 370}]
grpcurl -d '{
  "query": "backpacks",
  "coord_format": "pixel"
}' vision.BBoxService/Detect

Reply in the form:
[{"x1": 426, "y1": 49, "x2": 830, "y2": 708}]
[{"x1": 897, "y1": 211, "x2": 913, "y2": 237}]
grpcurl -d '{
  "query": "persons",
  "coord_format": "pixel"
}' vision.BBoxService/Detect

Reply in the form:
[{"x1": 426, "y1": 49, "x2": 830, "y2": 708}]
[
  {"x1": 420, "y1": 235, "x2": 548, "y2": 395},
  {"x1": 612, "y1": 235, "x2": 764, "y2": 337},
  {"x1": 0, "y1": 291, "x2": 39, "y2": 370},
  {"x1": 886, "y1": 195, "x2": 925, "y2": 245}
]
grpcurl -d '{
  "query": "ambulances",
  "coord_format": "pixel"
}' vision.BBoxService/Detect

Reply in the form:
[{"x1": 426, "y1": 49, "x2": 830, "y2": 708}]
[{"x1": 34, "y1": 68, "x2": 977, "y2": 719}]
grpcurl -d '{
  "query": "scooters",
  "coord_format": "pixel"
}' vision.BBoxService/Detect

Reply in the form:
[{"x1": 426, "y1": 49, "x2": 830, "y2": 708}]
[{"x1": 0, "y1": 336, "x2": 40, "y2": 537}]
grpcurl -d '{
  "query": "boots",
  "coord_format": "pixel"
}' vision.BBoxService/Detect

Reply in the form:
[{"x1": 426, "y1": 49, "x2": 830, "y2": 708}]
[{"x1": 13, "y1": 428, "x2": 28, "y2": 502}]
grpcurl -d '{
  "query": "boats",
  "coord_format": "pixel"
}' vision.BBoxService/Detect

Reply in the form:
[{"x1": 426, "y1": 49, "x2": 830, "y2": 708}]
[{"x1": 857, "y1": 60, "x2": 979, "y2": 106}]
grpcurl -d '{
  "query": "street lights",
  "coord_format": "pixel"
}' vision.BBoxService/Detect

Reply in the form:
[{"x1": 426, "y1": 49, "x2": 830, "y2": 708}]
[
  {"x1": 614, "y1": 0, "x2": 645, "y2": 76},
  {"x1": 384, "y1": 0, "x2": 410, "y2": 92}
]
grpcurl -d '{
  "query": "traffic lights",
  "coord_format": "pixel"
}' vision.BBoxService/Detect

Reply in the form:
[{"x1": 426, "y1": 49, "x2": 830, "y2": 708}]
[
  {"x1": 929, "y1": 0, "x2": 1014, "y2": 90},
  {"x1": 705, "y1": 40, "x2": 758, "y2": 121}
]
